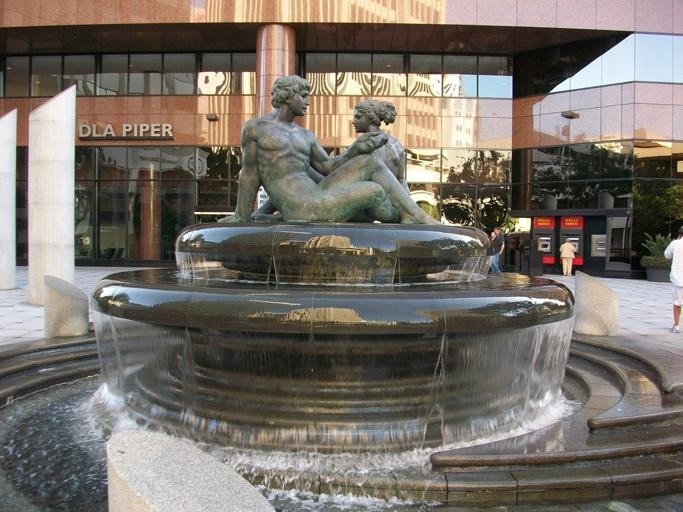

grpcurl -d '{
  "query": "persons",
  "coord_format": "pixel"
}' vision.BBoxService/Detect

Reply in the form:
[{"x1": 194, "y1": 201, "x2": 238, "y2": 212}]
[
  {"x1": 494, "y1": 227, "x2": 504, "y2": 272},
  {"x1": 662, "y1": 225, "x2": 683, "y2": 334},
  {"x1": 490, "y1": 232, "x2": 497, "y2": 273},
  {"x1": 251, "y1": 97, "x2": 407, "y2": 224},
  {"x1": 211, "y1": 73, "x2": 445, "y2": 225},
  {"x1": 558, "y1": 237, "x2": 576, "y2": 277}
]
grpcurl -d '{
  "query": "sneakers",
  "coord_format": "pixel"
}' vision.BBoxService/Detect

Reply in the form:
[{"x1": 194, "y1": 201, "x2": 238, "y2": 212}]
[{"x1": 672, "y1": 324, "x2": 679, "y2": 333}]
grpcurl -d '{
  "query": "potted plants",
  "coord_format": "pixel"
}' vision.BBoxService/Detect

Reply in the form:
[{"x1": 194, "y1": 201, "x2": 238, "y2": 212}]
[{"x1": 640, "y1": 231, "x2": 675, "y2": 282}]
